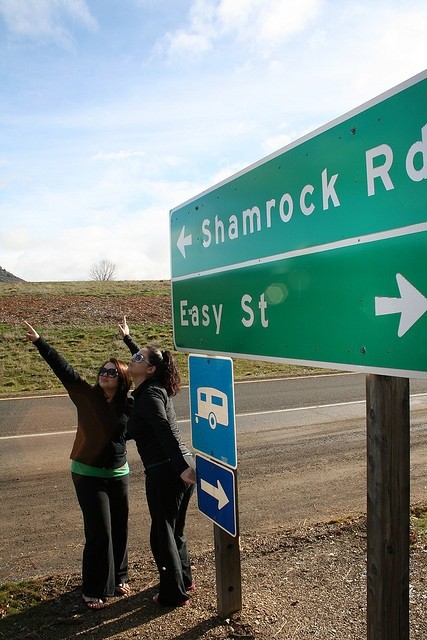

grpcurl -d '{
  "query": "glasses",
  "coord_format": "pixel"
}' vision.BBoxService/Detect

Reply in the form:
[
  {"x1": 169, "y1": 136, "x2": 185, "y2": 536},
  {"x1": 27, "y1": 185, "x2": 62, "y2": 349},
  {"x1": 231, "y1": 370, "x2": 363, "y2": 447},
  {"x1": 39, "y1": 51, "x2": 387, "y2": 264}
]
[
  {"x1": 98, "y1": 366, "x2": 119, "y2": 378},
  {"x1": 132, "y1": 353, "x2": 152, "y2": 366}
]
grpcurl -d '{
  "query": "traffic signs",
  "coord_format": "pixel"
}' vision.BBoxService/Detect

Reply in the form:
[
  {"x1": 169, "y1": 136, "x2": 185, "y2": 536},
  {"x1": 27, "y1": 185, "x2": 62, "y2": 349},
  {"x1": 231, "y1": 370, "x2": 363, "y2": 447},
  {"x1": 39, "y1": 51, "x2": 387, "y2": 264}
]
[
  {"x1": 169, "y1": 67, "x2": 427, "y2": 281},
  {"x1": 169, "y1": 221, "x2": 427, "y2": 382}
]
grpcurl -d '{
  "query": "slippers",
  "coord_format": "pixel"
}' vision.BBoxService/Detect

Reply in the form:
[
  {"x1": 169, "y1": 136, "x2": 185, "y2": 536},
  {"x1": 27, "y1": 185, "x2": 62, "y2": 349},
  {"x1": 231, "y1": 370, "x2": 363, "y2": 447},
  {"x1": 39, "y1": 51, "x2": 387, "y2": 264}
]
[
  {"x1": 153, "y1": 592, "x2": 189, "y2": 605},
  {"x1": 184, "y1": 583, "x2": 195, "y2": 592},
  {"x1": 83, "y1": 592, "x2": 105, "y2": 609},
  {"x1": 115, "y1": 581, "x2": 130, "y2": 595}
]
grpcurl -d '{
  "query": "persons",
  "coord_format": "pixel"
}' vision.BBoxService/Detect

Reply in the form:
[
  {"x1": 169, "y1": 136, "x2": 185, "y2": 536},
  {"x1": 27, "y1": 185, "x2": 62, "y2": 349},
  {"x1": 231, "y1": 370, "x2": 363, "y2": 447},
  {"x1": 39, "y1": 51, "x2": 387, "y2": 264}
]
[
  {"x1": 23, "y1": 320, "x2": 136, "y2": 610},
  {"x1": 118, "y1": 316, "x2": 196, "y2": 607}
]
